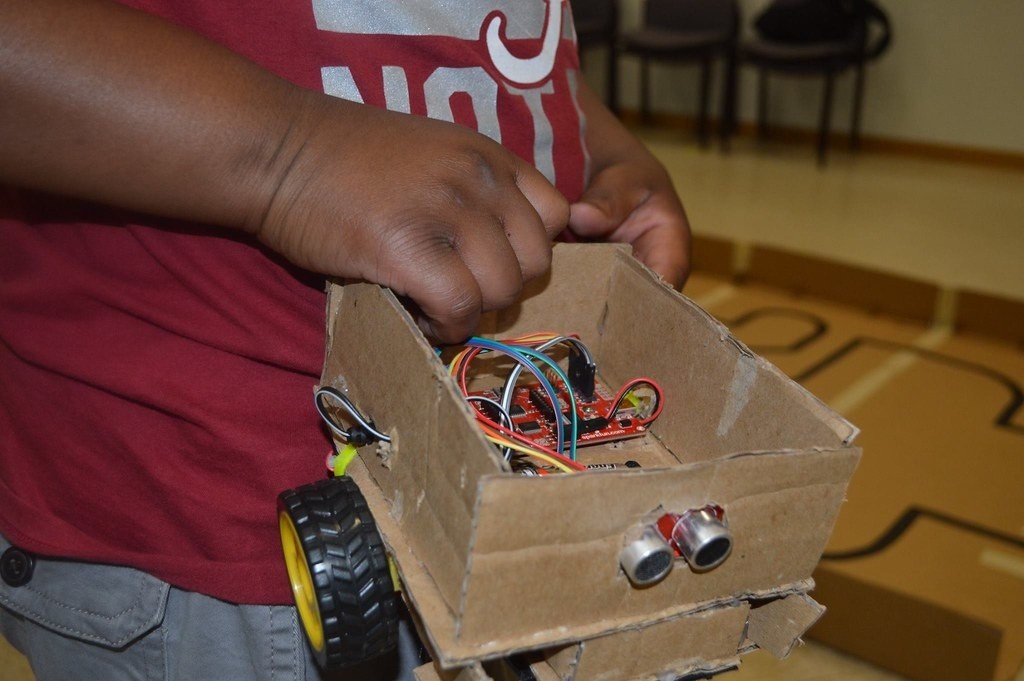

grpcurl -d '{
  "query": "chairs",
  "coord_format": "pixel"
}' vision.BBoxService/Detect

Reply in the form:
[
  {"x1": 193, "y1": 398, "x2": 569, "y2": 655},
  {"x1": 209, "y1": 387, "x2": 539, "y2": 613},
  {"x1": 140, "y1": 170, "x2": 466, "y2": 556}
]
[{"x1": 569, "y1": 0, "x2": 891, "y2": 168}]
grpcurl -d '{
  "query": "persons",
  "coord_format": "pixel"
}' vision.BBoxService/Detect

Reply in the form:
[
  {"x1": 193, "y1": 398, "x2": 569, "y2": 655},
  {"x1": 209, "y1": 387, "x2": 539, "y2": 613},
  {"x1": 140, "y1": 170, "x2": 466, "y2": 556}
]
[{"x1": 0, "y1": 0, "x2": 694, "y2": 681}]
